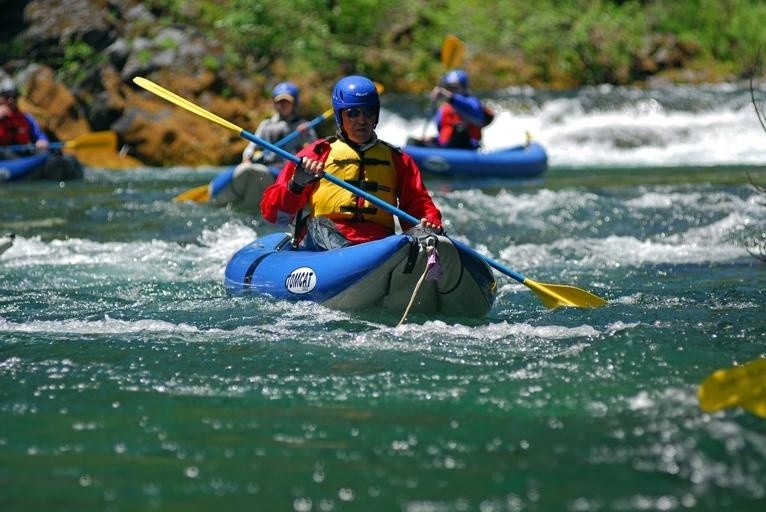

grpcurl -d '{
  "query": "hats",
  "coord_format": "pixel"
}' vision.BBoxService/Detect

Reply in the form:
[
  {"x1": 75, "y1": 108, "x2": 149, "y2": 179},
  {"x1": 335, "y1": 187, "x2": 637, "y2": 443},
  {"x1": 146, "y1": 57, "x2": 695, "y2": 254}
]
[{"x1": 274, "y1": 94, "x2": 295, "y2": 104}]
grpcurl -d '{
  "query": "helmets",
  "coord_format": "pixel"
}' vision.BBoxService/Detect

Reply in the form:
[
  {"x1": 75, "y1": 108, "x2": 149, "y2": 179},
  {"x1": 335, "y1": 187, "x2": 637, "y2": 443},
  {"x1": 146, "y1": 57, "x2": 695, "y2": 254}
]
[
  {"x1": 444, "y1": 70, "x2": 468, "y2": 87},
  {"x1": 332, "y1": 75, "x2": 380, "y2": 130},
  {"x1": 272, "y1": 83, "x2": 298, "y2": 98}
]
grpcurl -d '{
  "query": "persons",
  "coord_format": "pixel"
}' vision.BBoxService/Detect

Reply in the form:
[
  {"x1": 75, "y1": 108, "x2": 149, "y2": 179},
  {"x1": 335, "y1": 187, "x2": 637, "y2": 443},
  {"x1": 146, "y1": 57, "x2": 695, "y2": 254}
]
[
  {"x1": 407, "y1": 70, "x2": 494, "y2": 149},
  {"x1": 0, "y1": 80, "x2": 49, "y2": 160},
  {"x1": 242, "y1": 83, "x2": 319, "y2": 170},
  {"x1": 260, "y1": 75, "x2": 443, "y2": 251}
]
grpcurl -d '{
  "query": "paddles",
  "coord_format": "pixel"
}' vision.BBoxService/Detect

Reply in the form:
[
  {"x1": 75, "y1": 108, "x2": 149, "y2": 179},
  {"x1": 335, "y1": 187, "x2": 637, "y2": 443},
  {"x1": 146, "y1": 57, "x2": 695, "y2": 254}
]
[
  {"x1": 133, "y1": 76, "x2": 608, "y2": 308},
  {"x1": 0, "y1": 130, "x2": 117, "y2": 158},
  {"x1": 173, "y1": 82, "x2": 385, "y2": 202},
  {"x1": 420, "y1": 34, "x2": 465, "y2": 141}
]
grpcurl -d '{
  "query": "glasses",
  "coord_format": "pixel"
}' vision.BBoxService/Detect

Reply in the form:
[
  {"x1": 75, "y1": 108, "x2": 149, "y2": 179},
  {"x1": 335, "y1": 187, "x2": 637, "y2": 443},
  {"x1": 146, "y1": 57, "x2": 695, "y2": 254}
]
[{"x1": 345, "y1": 108, "x2": 376, "y2": 118}]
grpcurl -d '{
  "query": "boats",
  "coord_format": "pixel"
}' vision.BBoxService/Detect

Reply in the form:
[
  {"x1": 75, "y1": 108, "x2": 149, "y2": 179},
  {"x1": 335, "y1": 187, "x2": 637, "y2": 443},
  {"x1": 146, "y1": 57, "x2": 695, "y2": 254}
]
[
  {"x1": 401, "y1": 142, "x2": 547, "y2": 180},
  {"x1": 0, "y1": 149, "x2": 79, "y2": 184},
  {"x1": 223, "y1": 226, "x2": 495, "y2": 319},
  {"x1": 206, "y1": 163, "x2": 284, "y2": 209}
]
